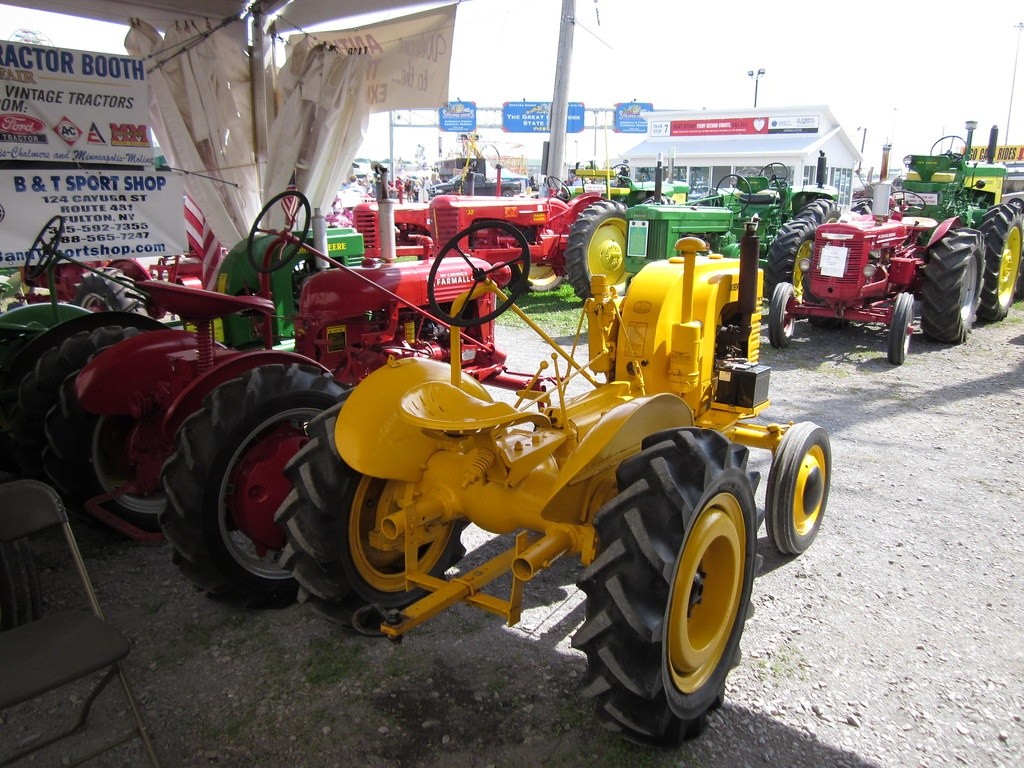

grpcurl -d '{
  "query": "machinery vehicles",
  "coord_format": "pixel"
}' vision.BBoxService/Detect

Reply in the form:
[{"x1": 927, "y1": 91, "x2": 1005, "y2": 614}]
[
  {"x1": 354, "y1": 161, "x2": 605, "y2": 292},
  {"x1": 0, "y1": 161, "x2": 831, "y2": 745},
  {"x1": 562, "y1": 149, "x2": 848, "y2": 302},
  {"x1": 765, "y1": 117, "x2": 1024, "y2": 363}
]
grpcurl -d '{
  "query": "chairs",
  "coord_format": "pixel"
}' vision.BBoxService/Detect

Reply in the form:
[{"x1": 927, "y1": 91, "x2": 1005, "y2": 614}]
[{"x1": 0, "y1": 470, "x2": 165, "y2": 768}]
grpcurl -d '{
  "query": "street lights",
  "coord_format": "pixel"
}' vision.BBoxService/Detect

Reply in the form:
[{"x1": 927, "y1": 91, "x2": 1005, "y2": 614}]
[
  {"x1": 857, "y1": 126, "x2": 867, "y2": 176},
  {"x1": 748, "y1": 68, "x2": 765, "y2": 108}
]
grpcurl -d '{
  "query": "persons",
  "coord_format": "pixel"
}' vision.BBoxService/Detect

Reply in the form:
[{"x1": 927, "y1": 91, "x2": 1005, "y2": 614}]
[
  {"x1": 349, "y1": 173, "x2": 381, "y2": 198},
  {"x1": 396, "y1": 176, "x2": 431, "y2": 202}
]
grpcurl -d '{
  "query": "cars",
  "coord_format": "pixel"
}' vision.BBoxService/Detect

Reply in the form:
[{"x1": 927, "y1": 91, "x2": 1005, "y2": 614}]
[{"x1": 1001, "y1": 192, "x2": 1024, "y2": 214}]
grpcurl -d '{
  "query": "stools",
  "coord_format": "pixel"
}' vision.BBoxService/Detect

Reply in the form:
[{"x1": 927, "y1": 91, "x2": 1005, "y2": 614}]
[{"x1": 396, "y1": 381, "x2": 552, "y2": 481}]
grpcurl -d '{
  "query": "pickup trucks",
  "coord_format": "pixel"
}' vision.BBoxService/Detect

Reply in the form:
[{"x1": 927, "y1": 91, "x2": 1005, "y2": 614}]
[{"x1": 426, "y1": 173, "x2": 521, "y2": 197}]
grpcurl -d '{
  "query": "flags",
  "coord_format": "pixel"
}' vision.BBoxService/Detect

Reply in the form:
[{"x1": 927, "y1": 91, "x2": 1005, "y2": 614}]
[
  {"x1": 281, "y1": 170, "x2": 297, "y2": 224},
  {"x1": 184, "y1": 195, "x2": 222, "y2": 291}
]
